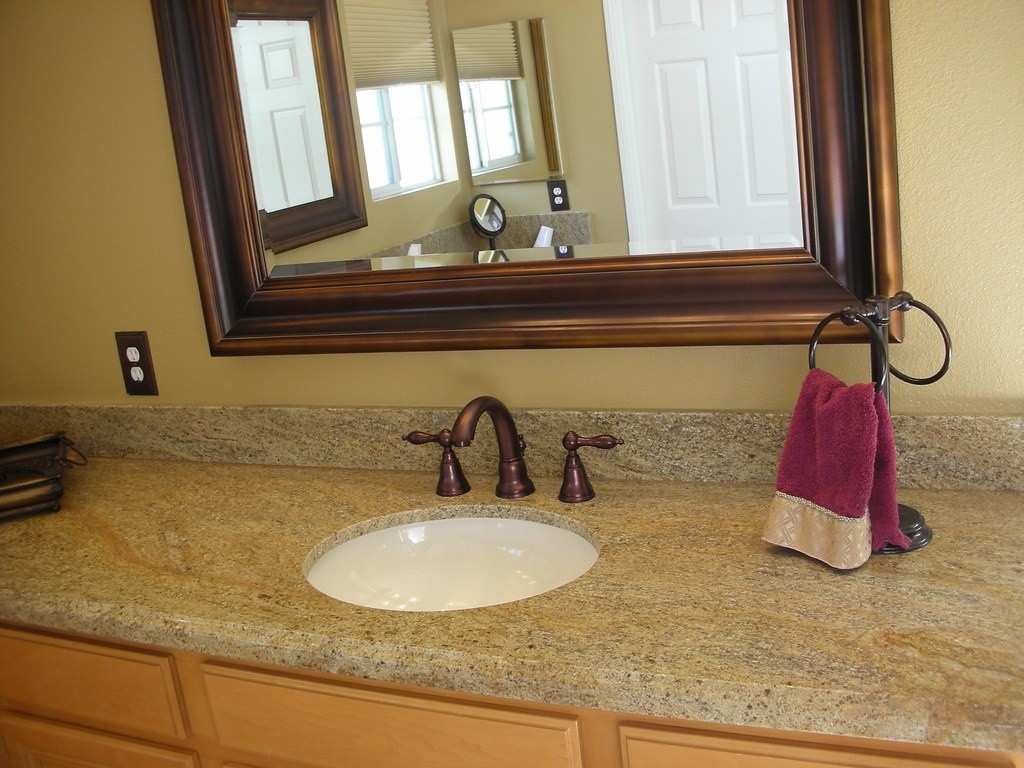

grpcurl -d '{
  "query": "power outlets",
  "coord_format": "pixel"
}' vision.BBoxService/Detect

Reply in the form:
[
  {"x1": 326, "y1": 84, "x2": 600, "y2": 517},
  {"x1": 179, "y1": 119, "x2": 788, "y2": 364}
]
[
  {"x1": 554, "y1": 244, "x2": 574, "y2": 260},
  {"x1": 115, "y1": 331, "x2": 159, "y2": 396},
  {"x1": 547, "y1": 180, "x2": 571, "y2": 211}
]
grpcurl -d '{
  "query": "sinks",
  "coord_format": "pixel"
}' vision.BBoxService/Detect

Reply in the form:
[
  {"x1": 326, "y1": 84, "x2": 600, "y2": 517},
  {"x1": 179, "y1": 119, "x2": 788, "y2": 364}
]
[{"x1": 301, "y1": 503, "x2": 605, "y2": 613}]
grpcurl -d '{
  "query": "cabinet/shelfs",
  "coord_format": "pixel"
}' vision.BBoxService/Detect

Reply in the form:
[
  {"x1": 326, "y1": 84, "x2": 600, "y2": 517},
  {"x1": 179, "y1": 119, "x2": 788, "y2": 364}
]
[{"x1": 0, "y1": 625, "x2": 1024, "y2": 768}]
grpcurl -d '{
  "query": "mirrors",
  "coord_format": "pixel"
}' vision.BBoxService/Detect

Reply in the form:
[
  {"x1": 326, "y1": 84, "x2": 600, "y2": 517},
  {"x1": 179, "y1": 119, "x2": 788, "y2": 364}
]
[
  {"x1": 469, "y1": 193, "x2": 507, "y2": 249},
  {"x1": 151, "y1": 0, "x2": 907, "y2": 358},
  {"x1": 227, "y1": 0, "x2": 368, "y2": 255}
]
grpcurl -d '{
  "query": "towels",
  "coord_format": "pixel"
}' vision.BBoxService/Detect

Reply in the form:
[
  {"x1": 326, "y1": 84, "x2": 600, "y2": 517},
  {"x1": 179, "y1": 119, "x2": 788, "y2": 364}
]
[{"x1": 762, "y1": 368, "x2": 913, "y2": 570}]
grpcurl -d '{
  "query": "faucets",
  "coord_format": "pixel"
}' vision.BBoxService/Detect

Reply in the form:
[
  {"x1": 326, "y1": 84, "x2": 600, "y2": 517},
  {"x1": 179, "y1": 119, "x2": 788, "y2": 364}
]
[{"x1": 451, "y1": 396, "x2": 536, "y2": 499}]
